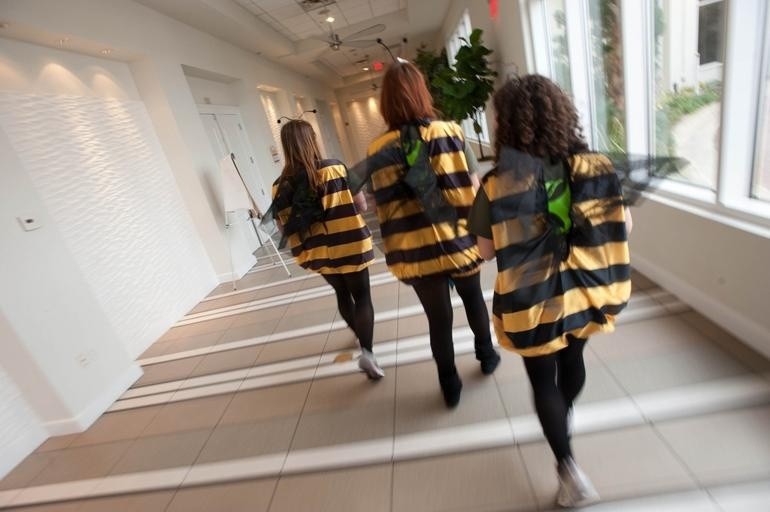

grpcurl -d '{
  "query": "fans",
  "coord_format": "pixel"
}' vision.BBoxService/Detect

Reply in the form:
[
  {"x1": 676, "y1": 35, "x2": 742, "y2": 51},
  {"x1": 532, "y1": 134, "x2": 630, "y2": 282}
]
[{"x1": 304, "y1": 0, "x2": 386, "y2": 60}]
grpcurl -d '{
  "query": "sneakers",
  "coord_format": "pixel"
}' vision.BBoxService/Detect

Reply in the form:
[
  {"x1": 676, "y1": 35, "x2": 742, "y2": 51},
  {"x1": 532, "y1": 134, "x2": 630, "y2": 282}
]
[
  {"x1": 479, "y1": 354, "x2": 502, "y2": 376},
  {"x1": 552, "y1": 461, "x2": 601, "y2": 510},
  {"x1": 358, "y1": 346, "x2": 386, "y2": 381},
  {"x1": 444, "y1": 379, "x2": 464, "y2": 410}
]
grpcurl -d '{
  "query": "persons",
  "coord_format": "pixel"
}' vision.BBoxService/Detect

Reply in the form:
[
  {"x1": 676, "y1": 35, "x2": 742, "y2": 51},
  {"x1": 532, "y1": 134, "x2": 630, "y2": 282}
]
[
  {"x1": 255, "y1": 108, "x2": 385, "y2": 380},
  {"x1": 344, "y1": 62, "x2": 501, "y2": 409},
  {"x1": 465, "y1": 74, "x2": 692, "y2": 508}
]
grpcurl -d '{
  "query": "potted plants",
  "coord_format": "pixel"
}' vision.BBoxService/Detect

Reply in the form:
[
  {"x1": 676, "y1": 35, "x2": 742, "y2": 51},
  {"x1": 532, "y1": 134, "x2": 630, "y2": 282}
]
[{"x1": 431, "y1": 28, "x2": 498, "y2": 179}]
